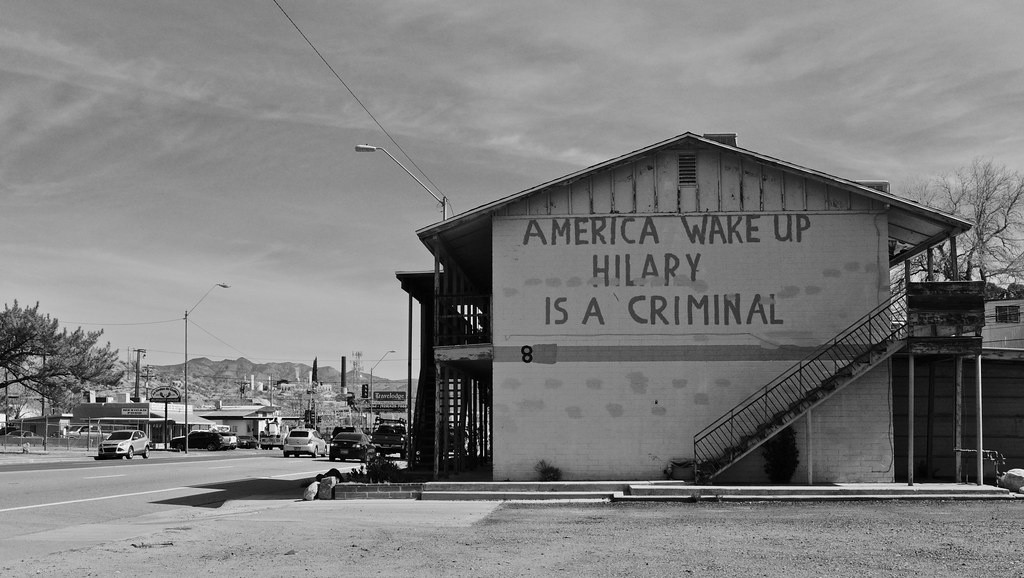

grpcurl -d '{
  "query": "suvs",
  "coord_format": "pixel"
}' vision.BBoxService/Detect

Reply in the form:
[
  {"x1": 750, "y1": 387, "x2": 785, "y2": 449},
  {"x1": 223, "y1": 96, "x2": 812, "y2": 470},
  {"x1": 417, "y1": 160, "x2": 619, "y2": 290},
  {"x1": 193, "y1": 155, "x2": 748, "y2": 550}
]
[
  {"x1": 170, "y1": 430, "x2": 226, "y2": 452},
  {"x1": 370, "y1": 425, "x2": 408, "y2": 460}
]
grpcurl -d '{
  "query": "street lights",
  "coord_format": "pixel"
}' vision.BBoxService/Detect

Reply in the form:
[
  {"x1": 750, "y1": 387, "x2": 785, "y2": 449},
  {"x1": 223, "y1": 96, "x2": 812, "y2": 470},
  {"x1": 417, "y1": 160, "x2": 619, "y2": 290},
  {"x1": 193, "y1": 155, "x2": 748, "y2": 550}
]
[
  {"x1": 181, "y1": 283, "x2": 231, "y2": 454},
  {"x1": 371, "y1": 350, "x2": 398, "y2": 435},
  {"x1": 354, "y1": 143, "x2": 448, "y2": 221}
]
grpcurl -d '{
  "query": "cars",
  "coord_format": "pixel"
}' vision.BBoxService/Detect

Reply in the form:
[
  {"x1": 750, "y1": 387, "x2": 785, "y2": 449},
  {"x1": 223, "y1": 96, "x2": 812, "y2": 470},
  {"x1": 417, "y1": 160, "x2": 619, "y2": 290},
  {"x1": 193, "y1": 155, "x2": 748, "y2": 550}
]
[
  {"x1": 329, "y1": 432, "x2": 369, "y2": 463},
  {"x1": 97, "y1": 430, "x2": 150, "y2": 460},
  {"x1": 4, "y1": 431, "x2": 40, "y2": 438},
  {"x1": 66, "y1": 424, "x2": 101, "y2": 438},
  {"x1": 283, "y1": 427, "x2": 327, "y2": 458}
]
